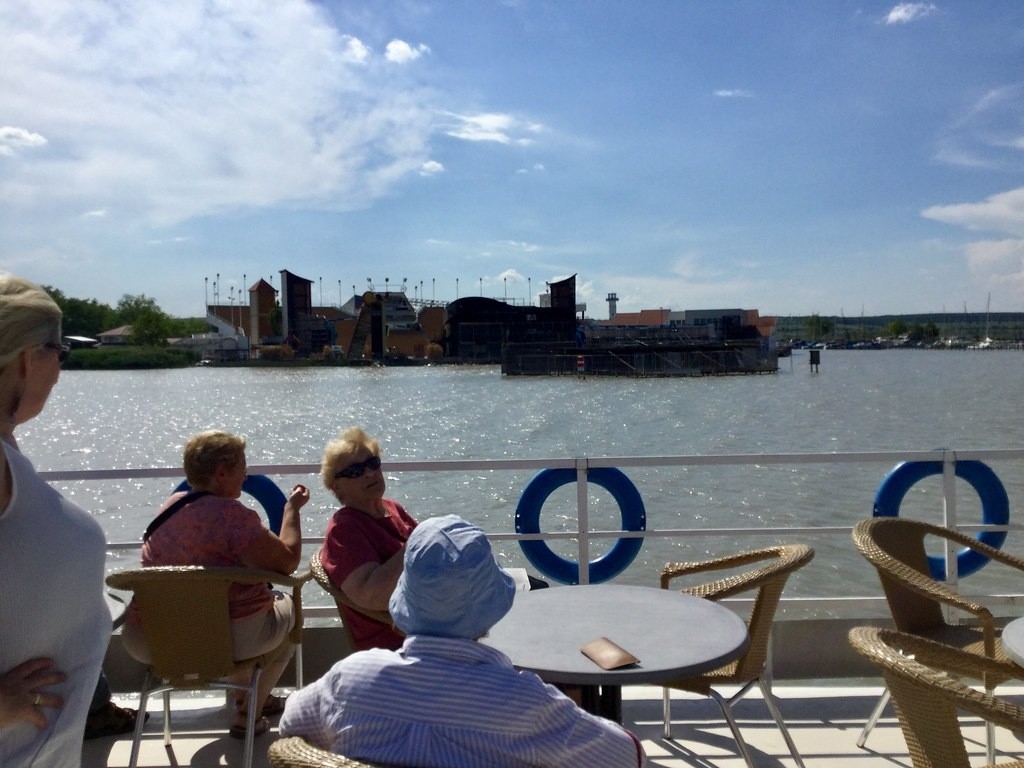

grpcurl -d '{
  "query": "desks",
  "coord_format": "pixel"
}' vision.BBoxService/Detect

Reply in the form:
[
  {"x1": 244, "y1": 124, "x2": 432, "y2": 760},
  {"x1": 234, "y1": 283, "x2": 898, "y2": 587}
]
[{"x1": 477, "y1": 586, "x2": 750, "y2": 724}]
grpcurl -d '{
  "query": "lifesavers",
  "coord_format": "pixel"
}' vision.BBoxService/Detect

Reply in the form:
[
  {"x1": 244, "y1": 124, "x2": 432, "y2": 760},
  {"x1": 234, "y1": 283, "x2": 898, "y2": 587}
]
[
  {"x1": 172, "y1": 471, "x2": 289, "y2": 537},
  {"x1": 514, "y1": 468, "x2": 646, "y2": 586},
  {"x1": 873, "y1": 446, "x2": 1011, "y2": 582}
]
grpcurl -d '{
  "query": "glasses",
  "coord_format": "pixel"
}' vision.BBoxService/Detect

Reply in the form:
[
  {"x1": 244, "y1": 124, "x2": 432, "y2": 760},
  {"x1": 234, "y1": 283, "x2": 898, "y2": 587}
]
[
  {"x1": 233, "y1": 464, "x2": 250, "y2": 477},
  {"x1": 334, "y1": 456, "x2": 381, "y2": 479},
  {"x1": 45, "y1": 342, "x2": 71, "y2": 367}
]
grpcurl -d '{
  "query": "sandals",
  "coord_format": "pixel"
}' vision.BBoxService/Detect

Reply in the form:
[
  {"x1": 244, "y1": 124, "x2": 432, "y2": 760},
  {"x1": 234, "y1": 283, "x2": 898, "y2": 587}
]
[
  {"x1": 237, "y1": 693, "x2": 288, "y2": 716},
  {"x1": 229, "y1": 708, "x2": 268, "y2": 739}
]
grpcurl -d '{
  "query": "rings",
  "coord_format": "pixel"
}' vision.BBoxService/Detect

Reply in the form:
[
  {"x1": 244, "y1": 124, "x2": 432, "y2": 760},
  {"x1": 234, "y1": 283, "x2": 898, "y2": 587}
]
[{"x1": 35, "y1": 691, "x2": 44, "y2": 707}]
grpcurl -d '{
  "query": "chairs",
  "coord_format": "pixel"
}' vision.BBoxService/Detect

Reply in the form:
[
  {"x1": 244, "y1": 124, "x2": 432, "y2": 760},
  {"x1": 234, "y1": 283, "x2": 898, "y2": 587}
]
[
  {"x1": 105, "y1": 565, "x2": 317, "y2": 768},
  {"x1": 851, "y1": 517, "x2": 1024, "y2": 767},
  {"x1": 659, "y1": 543, "x2": 816, "y2": 768},
  {"x1": 309, "y1": 550, "x2": 406, "y2": 653},
  {"x1": 847, "y1": 625, "x2": 1024, "y2": 768}
]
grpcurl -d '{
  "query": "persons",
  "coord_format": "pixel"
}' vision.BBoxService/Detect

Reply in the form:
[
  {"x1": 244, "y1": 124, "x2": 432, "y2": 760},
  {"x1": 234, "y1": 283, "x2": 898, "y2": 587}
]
[
  {"x1": 279, "y1": 511, "x2": 649, "y2": 767},
  {"x1": 139, "y1": 431, "x2": 311, "y2": 742},
  {"x1": 1, "y1": 279, "x2": 151, "y2": 768},
  {"x1": 315, "y1": 430, "x2": 420, "y2": 652}
]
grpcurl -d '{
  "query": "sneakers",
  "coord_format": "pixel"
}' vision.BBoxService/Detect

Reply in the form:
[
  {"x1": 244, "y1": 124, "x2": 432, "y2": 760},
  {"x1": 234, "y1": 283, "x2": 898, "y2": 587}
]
[{"x1": 85, "y1": 702, "x2": 150, "y2": 740}]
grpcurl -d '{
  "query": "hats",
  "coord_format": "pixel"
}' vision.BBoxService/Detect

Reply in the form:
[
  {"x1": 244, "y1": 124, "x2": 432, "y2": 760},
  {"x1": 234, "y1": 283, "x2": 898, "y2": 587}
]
[{"x1": 387, "y1": 513, "x2": 517, "y2": 639}]
